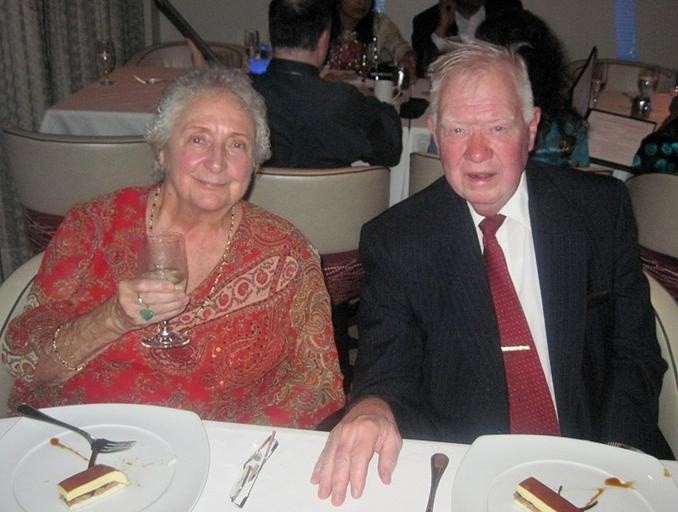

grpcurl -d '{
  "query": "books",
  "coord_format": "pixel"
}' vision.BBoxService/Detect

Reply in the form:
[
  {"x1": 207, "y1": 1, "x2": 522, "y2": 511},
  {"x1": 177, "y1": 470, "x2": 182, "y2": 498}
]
[{"x1": 564, "y1": 46, "x2": 657, "y2": 172}]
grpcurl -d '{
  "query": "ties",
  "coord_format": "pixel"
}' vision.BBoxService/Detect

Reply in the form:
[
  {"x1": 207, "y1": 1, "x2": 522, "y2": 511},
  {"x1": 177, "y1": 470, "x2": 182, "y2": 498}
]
[{"x1": 478, "y1": 213, "x2": 562, "y2": 436}]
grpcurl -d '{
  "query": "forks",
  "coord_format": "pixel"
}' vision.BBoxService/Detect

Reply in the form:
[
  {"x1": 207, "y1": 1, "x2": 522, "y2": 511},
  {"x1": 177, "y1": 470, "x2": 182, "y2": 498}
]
[{"x1": 17, "y1": 405, "x2": 135, "y2": 451}]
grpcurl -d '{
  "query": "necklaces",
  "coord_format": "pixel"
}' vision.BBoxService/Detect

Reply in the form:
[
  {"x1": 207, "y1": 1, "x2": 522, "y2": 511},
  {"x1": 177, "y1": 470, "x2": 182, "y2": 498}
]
[{"x1": 149, "y1": 185, "x2": 235, "y2": 338}]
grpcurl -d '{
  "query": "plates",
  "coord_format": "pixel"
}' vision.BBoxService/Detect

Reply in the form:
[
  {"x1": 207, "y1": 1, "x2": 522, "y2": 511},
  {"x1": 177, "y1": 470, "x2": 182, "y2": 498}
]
[
  {"x1": 0, "y1": 402, "x2": 211, "y2": 512},
  {"x1": 452, "y1": 432, "x2": 678, "y2": 512}
]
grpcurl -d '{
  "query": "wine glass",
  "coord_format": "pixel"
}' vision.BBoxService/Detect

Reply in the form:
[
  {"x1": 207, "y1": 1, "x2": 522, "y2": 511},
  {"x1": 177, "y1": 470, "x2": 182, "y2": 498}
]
[
  {"x1": 357, "y1": 49, "x2": 371, "y2": 82},
  {"x1": 592, "y1": 59, "x2": 608, "y2": 109},
  {"x1": 638, "y1": 63, "x2": 658, "y2": 99},
  {"x1": 138, "y1": 232, "x2": 192, "y2": 348},
  {"x1": 245, "y1": 30, "x2": 261, "y2": 61},
  {"x1": 97, "y1": 40, "x2": 116, "y2": 85}
]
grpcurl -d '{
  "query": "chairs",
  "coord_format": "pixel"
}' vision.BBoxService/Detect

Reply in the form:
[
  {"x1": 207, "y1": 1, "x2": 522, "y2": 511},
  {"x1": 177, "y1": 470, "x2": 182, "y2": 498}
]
[
  {"x1": 621, "y1": 174, "x2": 678, "y2": 260},
  {"x1": 567, "y1": 57, "x2": 676, "y2": 92},
  {"x1": 126, "y1": 39, "x2": 250, "y2": 70},
  {"x1": 408, "y1": 152, "x2": 614, "y2": 212},
  {"x1": 244, "y1": 165, "x2": 391, "y2": 394},
  {"x1": 644, "y1": 273, "x2": 677, "y2": 456},
  {"x1": 0, "y1": 250, "x2": 42, "y2": 419},
  {"x1": 2, "y1": 127, "x2": 153, "y2": 261}
]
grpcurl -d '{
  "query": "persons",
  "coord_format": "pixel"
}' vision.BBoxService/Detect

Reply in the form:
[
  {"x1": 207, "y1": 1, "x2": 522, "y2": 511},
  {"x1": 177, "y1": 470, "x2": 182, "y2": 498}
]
[
  {"x1": 186, "y1": 0, "x2": 402, "y2": 169},
  {"x1": 631, "y1": 98, "x2": 678, "y2": 176},
  {"x1": 322, "y1": 0, "x2": 417, "y2": 85},
  {"x1": 412, "y1": 0, "x2": 524, "y2": 78},
  {"x1": 311, "y1": 36, "x2": 675, "y2": 508},
  {"x1": 428, "y1": 11, "x2": 592, "y2": 167},
  {"x1": 0, "y1": 65, "x2": 346, "y2": 429}
]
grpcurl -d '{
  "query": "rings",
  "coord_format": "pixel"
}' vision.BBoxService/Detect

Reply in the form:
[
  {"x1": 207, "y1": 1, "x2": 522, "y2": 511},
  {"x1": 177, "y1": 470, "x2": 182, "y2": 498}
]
[
  {"x1": 140, "y1": 306, "x2": 154, "y2": 321},
  {"x1": 137, "y1": 290, "x2": 147, "y2": 307}
]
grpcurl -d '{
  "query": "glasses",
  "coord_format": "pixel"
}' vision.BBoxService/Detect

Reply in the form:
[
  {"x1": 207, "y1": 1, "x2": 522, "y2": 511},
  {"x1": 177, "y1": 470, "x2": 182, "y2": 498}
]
[{"x1": 228, "y1": 431, "x2": 282, "y2": 510}]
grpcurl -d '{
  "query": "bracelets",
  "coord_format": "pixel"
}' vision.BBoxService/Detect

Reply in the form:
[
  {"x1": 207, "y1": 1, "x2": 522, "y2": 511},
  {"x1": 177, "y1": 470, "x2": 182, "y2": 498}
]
[{"x1": 52, "y1": 324, "x2": 87, "y2": 373}]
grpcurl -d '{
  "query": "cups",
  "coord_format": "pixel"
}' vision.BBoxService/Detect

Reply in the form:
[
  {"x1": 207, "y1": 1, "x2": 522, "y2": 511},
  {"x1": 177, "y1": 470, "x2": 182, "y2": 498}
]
[{"x1": 374, "y1": 80, "x2": 401, "y2": 104}]
